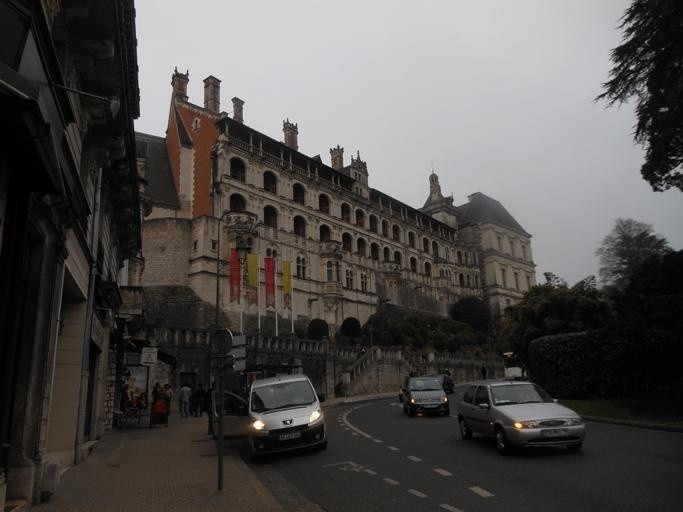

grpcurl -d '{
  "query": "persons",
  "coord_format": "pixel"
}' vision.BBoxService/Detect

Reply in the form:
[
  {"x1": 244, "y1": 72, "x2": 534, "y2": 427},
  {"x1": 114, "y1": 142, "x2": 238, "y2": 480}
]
[
  {"x1": 407, "y1": 364, "x2": 452, "y2": 390},
  {"x1": 480, "y1": 362, "x2": 487, "y2": 379},
  {"x1": 120, "y1": 377, "x2": 217, "y2": 418}
]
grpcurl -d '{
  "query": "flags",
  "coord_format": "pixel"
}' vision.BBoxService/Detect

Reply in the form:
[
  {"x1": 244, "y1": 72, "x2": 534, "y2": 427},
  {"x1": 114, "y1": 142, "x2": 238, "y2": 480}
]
[
  {"x1": 229, "y1": 248, "x2": 241, "y2": 306},
  {"x1": 242, "y1": 251, "x2": 260, "y2": 313},
  {"x1": 263, "y1": 258, "x2": 278, "y2": 312},
  {"x1": 283, "y1": 261, "x2": 292, "y2": 313}
]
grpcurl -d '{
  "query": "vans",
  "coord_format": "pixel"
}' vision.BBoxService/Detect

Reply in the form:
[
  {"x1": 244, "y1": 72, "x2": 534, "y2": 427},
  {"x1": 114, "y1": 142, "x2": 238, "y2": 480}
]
[{"x1": 208, "y1": 371, "x2": 330, "y2": 466}]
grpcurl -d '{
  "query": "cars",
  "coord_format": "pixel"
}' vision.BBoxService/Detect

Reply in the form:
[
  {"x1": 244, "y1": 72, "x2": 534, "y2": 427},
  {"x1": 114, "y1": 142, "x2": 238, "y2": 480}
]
[
  {"x1": 399, "y1": 370, "x2": 451, "y2": 418},
  {"x1": 456, "y1": 379, "x2": 588, "y2": 458}
]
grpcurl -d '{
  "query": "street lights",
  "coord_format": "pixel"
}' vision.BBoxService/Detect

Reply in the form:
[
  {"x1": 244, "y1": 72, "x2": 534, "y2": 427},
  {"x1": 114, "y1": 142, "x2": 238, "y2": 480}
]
[{"x1": 212, "y1": 207, "x2": 256, "y2": 493}]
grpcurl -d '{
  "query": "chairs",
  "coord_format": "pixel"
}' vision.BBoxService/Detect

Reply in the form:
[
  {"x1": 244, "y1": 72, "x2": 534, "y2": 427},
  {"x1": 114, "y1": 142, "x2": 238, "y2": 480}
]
[{"x1": 115, "y1": 409, "x2": 129, "y2": 431}]
